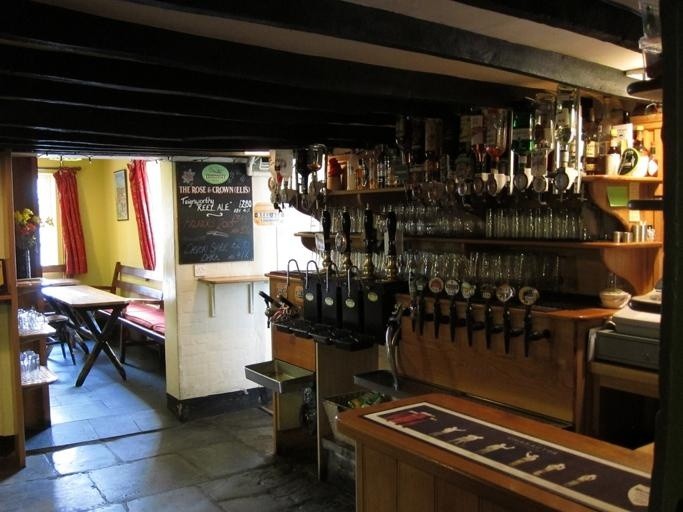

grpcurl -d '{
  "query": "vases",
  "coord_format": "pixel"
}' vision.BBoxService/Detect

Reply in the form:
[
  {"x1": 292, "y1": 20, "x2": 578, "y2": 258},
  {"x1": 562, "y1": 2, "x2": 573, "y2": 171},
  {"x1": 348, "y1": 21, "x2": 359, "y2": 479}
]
[{"x1": 17, "y1": 248, "x2": 32, "y2": 278}]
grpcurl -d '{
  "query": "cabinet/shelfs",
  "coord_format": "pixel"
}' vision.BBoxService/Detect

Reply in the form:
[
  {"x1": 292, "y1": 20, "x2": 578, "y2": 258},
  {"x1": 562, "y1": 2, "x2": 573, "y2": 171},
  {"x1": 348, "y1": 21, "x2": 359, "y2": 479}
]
[
  {"x1": 296, "y1": 175, "x2": 663, "y2": 321},
  {"x1": 18, "y1": 279, "x2": 58, "y2": 388}
]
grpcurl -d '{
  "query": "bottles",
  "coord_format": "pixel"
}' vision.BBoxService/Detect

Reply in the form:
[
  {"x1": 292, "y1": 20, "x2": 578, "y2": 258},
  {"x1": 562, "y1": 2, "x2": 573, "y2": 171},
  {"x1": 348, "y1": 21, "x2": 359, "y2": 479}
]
[
  {"x1": 403, "y1": 243, "x2": 562, "y2": 304},
  {"x1": 267, "y1": 149, "x2": 324, "y2": 195},
  {"x1": 324, "y1": 248, "x2": 403, "y2": 279},
  {"x1": 321, "y1": 204, "x2": 405, "y2": 234},
  {"x1": 326, "y1": 82, "x2": 661, "y2": 192}
]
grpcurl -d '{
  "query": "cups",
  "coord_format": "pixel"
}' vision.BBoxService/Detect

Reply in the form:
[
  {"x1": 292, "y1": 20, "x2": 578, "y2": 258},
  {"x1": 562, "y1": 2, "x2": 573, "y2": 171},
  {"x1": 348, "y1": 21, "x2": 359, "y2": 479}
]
[
  {"x1": 405, "y1": 193, "x2": 592, "y2": 241},
  {"x1": 17, "y1": 308, "x2": 48, "y2": 333},
  {"x1": 19, "y1": 350, "x2": 39, "y2": 382}
]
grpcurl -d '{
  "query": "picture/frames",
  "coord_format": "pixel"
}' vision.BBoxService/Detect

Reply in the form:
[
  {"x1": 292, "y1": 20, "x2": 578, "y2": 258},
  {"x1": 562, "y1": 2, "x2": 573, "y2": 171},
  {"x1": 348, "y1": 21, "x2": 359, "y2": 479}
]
[{"x1": 113, "y1": 169, "x2": 129, "y2": 221}]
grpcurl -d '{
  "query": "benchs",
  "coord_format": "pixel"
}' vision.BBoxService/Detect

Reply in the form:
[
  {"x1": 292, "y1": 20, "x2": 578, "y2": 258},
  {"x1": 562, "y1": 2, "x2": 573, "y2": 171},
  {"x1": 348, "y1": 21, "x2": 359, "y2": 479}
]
[{"x1": 85, "y1": 262, "x2": 164, "y2": 363}]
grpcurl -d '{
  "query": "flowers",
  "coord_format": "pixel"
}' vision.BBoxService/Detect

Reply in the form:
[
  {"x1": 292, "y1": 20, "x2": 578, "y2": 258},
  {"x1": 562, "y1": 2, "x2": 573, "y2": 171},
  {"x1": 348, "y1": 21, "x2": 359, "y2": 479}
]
[{"x1": 14, "y1": 208, "x2": 55, "y2": 248}]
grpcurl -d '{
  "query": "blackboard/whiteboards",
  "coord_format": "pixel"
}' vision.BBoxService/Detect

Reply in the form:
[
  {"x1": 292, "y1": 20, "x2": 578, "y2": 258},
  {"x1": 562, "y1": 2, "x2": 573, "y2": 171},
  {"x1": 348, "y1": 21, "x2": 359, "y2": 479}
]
[{"x1": 172, "y1": 160, "x2": 254, "y2": 265}]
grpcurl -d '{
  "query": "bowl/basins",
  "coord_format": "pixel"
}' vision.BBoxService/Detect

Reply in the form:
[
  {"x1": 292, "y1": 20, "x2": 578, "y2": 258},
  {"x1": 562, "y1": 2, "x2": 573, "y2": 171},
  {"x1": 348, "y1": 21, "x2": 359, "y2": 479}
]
[{"x1": 598, "y1": 290, "x2": 631, "y2": 310}]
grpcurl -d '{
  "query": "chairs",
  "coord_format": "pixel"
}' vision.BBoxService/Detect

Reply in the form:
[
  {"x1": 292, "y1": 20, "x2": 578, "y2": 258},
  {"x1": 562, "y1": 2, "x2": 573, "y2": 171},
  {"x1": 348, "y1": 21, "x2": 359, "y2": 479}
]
[{"x1": 43, "y1": 310, "x2": 76, "y2": 367}]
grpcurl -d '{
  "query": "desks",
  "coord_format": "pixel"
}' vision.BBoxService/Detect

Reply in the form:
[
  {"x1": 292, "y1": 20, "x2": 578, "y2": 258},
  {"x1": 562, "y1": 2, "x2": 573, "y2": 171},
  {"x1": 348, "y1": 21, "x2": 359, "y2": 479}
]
[
  {"x1": 41, "y1": 285, "x2": 129, "y2": 386},
  {"x1": 336, "y1": 390, "x2": 655, "y2": 511}
]
